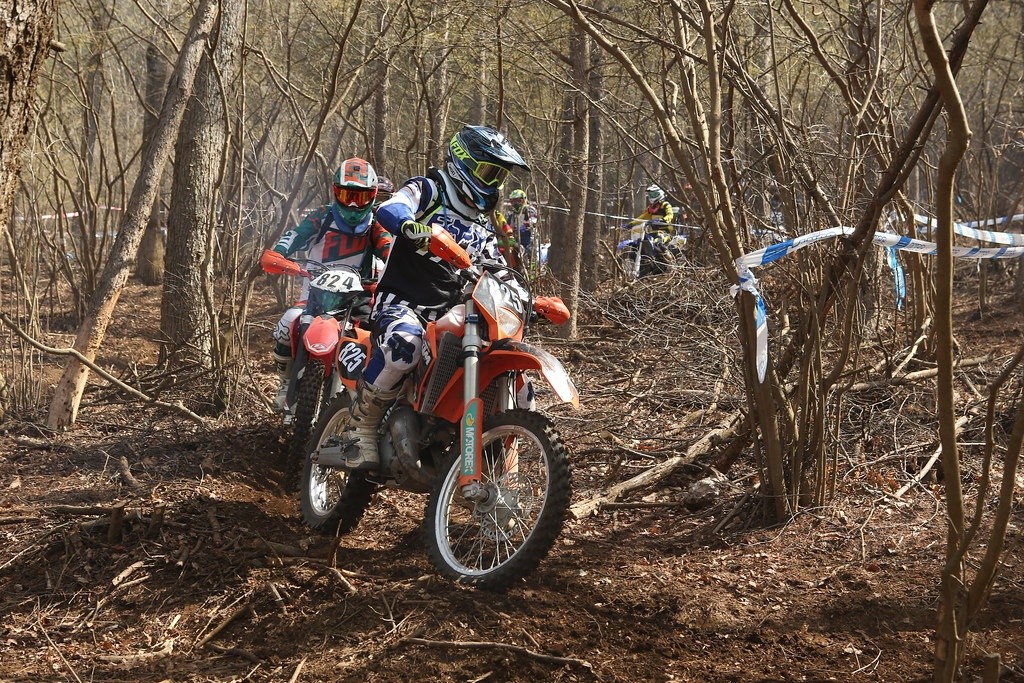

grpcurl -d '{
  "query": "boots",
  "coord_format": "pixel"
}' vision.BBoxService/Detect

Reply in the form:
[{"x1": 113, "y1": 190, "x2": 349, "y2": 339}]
[
  {"x1": 272, "y1": 351, "x2": 293, "y2": 411},
  {"x1": 662, "y1": 250, "x2": 673, "y2": 261},
  {"x1": 339, "y1": 376, "x2": 410, "y2": 468}
]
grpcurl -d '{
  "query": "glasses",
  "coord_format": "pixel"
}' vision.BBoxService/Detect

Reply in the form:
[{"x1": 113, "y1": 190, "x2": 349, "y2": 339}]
[{"x1": 450, "y1": 132, "x2": 510, "y2": 189}]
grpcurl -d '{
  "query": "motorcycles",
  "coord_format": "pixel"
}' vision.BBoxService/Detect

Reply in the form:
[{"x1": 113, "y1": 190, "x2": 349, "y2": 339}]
[
  {"x1": 507, "y1": 214, "x2": 548, "y2": 270},
  {"x1": 257, "y1": 249, "x2": 380, "y2": 487},
  {"x1": 294, "y1": 220, "x2": 583, "y2": 594},
  {"x1": 616, "y1": 218, "x2": 690, "y2": 284}
]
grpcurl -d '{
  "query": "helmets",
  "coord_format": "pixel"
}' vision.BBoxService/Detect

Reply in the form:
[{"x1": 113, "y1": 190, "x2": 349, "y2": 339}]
[
  {"x1": 333, "y1": 158, "x2": 378, "y2": 227},
  {"x1": 443, "y1": 125, "x2": 532, "y2": 213},
  {"x1": 646, "y1": 185, "x2": 667, "y2": 204},
  {"x1": 372, "y1": 177, "x2": 395, "y2": 212}
]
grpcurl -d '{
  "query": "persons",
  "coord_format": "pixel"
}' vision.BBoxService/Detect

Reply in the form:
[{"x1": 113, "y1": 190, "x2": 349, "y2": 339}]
[
  {"x1": 503, "y1": 188, "x2": 538, "y2": 274},
  {"x1": 346, "y1": 124, "x2": 553, "y2": 471},
  {"x1": 623, "y1": 183, "x2": 675, "y2": 271},
  {"x1": 263, "y1": 159, "x2": 393, "y2": 414}
]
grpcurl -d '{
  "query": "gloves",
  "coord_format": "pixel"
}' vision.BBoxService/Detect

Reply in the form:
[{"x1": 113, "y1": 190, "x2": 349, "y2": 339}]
[
  {"x1": 621, "y1": 224, "x2": 629, "y2": 229},
  {"x1": 401, "y1": 220, "x2": 432, "y2": 252},
  {"x1": 653, "y1": 218, "x2": 660, "y2": 224}
]
